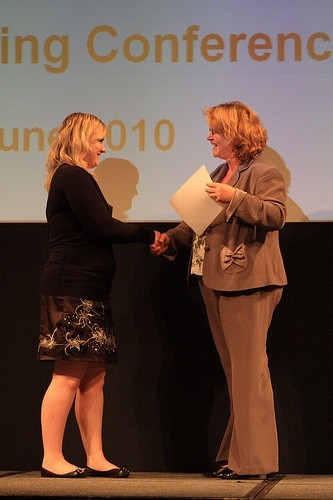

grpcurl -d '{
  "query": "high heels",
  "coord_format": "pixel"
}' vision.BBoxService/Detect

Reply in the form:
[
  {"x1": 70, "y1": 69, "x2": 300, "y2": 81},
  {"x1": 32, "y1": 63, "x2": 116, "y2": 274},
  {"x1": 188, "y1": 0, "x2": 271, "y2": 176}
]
[
  {"x1": 221, "y1": 468, "x2": 277, "y2": 479},
  {"x1": 204, "y1": 463, "x2": 226, "y2": 477}
]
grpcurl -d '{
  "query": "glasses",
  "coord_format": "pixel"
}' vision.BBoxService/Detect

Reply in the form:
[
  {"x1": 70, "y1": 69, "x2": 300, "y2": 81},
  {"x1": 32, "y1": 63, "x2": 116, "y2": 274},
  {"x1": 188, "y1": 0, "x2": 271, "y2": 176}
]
[{"x1": 208, "y1": 126, "x2": 218, "y2": 134}]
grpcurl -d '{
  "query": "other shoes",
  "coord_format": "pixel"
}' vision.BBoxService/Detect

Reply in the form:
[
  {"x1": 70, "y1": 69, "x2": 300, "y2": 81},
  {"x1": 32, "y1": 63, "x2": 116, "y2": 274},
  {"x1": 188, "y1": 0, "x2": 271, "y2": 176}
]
[
  {"x1": 41, "y1": 466, "x2": 100, "y2": 478},
  {"x1": 85, "y1": 465, "x2": 130, "y2": 478}
]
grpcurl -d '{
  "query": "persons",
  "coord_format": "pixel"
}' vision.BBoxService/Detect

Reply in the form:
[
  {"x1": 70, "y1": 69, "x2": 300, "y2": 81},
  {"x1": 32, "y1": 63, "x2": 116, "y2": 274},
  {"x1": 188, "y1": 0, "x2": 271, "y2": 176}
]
[
  {"x1": 36, "y1": 113, "x2": 164, "y2": 478},
  {"x1": 150, "y1": 100, "x2": 288, "y2": 479}
]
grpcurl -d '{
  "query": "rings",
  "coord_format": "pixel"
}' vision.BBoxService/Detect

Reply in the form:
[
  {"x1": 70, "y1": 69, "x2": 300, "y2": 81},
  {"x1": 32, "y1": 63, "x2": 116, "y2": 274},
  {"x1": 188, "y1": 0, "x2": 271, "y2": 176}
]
[{"x1": 212, "y1": 193, "x2": 215, "y2": 198}]
